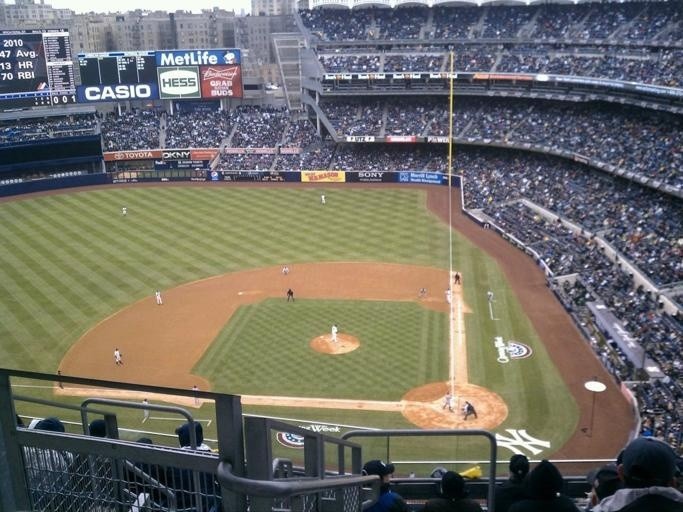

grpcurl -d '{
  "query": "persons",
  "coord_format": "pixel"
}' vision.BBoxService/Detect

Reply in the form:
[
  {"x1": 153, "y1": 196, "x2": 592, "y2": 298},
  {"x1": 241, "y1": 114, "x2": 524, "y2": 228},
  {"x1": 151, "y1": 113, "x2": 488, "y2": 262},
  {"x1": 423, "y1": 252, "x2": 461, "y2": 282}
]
[
  {"x1": 193, "y1": 386, "x2": 199, "y2": 403},
  {"x1": 298, "y1": 0, "x2": 683, "y2": 464},
  {"x1": 358, "y1": 437, "x2": 683, "y2": 512},
  {"x1": 114, "y1": 348, "x2": 123, "y2": 366},
  {"x1": 19, "y1": 413, "x2": 220, "y2": 512},
  {"x1": 283, "y1": 266, "x2": 294, "y2": 300},
  {"x1": 156, "y1": 289, "x2": 162, "y2": 305},
  {"x1": 0, "y1": 102, "x2": 318, "y2": 178},
  {"x1": 122, "y1": 206, "x2": 127, "y2": 215},
  {"x1": 143, "y1": 399, "x2": 149, "y2": 417},
  {"x1": 57, "y1": 370, "x2": 63, "y2": 389}
]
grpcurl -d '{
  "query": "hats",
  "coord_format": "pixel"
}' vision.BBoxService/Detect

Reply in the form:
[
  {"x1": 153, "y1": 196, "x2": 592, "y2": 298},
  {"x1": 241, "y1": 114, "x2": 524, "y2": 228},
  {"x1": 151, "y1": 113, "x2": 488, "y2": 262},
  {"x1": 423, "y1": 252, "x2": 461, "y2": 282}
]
[
  {"x1": 622, "y1": 436, "x2": 678, "y2": 481},
  {"x1": 587, "y1": 465, "x2": 620, "y2": 500},
  {"x1": 361, "y1": 460, "x2": 395, "y2": 476},
  {"x1": 175, "y1": 422, "x2": 203, "y2": 444}
]
